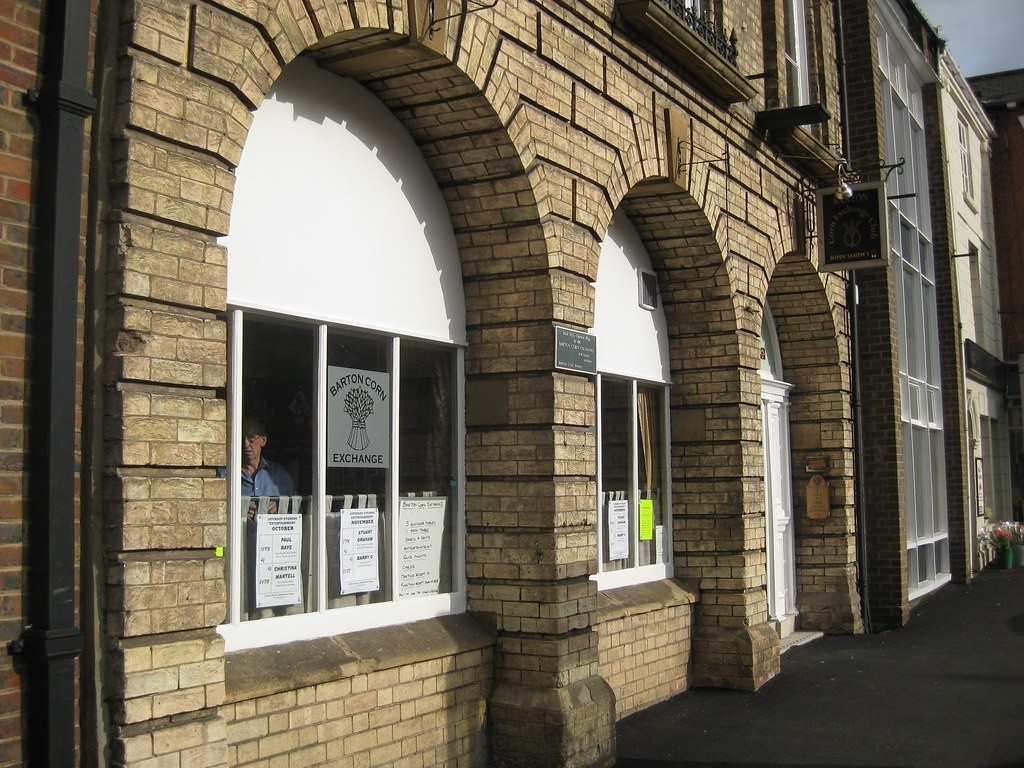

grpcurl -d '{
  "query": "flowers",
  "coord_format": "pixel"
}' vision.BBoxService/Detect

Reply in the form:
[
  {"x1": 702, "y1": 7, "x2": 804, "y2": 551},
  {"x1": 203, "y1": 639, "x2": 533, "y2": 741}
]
[
  {"x1": 980, "y1": 522, "x2": 1012, "y2": 550},
  {"x1": 1004, "y1": 521, "x2": 1024, "y2": 545}
]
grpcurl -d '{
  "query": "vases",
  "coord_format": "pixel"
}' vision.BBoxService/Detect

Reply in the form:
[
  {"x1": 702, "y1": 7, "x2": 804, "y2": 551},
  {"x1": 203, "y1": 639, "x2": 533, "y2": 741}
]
[
  {"x1": 1013, "y1": 545, "x2": 1024, "y2": 566},
  {"x1": 998, "y1": 548, "x2": 1013, "y2": 570}
]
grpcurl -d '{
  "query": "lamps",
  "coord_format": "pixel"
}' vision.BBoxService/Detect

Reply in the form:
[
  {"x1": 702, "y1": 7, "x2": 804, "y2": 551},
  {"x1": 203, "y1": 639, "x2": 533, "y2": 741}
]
[{"x1": 834, "y1": 163, "x2": 859, "y2": 200}]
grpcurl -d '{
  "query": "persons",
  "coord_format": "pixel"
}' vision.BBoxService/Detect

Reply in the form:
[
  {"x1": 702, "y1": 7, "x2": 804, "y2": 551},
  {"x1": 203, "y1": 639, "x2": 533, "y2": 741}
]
[{"x1": 242, "y1": 413, "x2": 298, "y2": 524}]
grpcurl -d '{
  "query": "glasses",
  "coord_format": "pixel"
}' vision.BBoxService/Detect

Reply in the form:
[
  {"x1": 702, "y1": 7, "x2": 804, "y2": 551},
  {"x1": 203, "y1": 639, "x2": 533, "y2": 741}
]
[{"x1": 242, "y1": 432, "x2": 267, "y2": 441}]
